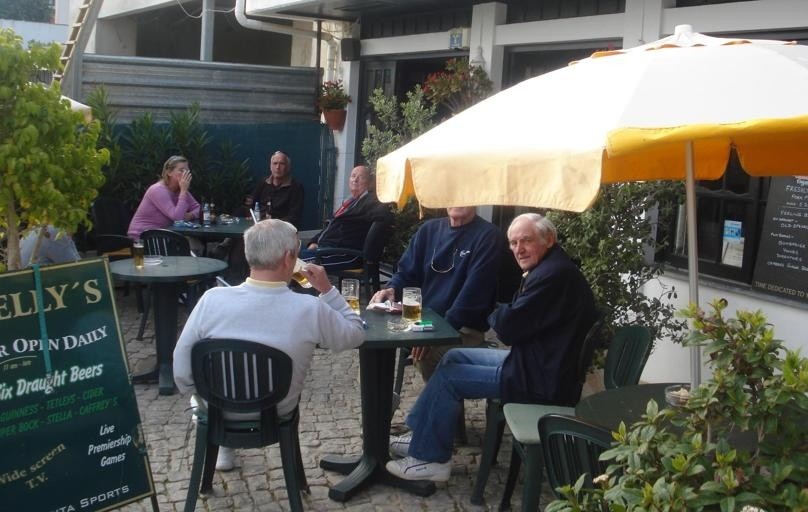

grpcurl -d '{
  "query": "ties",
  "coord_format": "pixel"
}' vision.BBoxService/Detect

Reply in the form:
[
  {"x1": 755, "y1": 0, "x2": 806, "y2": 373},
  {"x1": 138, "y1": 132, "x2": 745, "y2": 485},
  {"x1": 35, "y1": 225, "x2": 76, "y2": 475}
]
[{"x1": 333, "y1": 196, "x2": 356, "y2": 219}]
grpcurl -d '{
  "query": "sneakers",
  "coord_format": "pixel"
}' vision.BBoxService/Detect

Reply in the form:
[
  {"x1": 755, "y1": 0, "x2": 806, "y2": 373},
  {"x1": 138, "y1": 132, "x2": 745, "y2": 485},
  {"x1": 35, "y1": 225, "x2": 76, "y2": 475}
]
[
  {"x1": 216, "y1": 448, "x2": 235, "y2": 470},
  {"x1": 388, "y1": 436, "x2": 413, "y2": 456},
  {"x1": 386, "y1": 458, "x2": 451, "y2": 481}
]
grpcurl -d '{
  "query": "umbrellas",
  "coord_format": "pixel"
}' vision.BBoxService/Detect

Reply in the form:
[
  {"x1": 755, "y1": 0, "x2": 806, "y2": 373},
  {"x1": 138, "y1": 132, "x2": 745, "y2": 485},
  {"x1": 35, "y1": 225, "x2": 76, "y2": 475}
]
[{"x1": 375, "y1": 24, "x2": 808, "y2": 394}]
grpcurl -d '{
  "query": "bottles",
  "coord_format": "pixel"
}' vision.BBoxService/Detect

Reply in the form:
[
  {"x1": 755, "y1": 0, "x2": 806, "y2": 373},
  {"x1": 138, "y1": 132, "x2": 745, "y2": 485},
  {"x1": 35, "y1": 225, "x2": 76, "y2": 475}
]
[{"x1": 203, "y1": 201, "x2": 272, "y2": 227}]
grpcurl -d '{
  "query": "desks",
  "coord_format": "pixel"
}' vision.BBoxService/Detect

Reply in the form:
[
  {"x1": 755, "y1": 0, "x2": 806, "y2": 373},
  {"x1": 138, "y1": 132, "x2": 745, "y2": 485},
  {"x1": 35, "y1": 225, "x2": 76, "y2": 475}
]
[
  {"x1": 575, "y1": 383, "x2": 788, "y2": 463},
  {"x1": 107, "y1": 256, "x2": 229, "y2": 395},
  {"x1": 316, "y1": 304, "x2": 462, "y2": 502}
]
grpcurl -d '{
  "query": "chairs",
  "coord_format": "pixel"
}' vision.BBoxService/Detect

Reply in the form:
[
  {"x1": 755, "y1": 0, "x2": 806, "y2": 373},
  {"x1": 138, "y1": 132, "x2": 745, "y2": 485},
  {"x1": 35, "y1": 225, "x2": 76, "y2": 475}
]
[
  {"x1": 538, "y1": 412, "x2": 619, "y2": 501},
  {"x1": 184, "y1": 338, "x2": 311, "y2": 512},
  {"x1": 498, "y1": 324, "x2": 655, "y2": 511},
  {"x1": 471, "y1": 307, "x2": 604, "y2": 505}
]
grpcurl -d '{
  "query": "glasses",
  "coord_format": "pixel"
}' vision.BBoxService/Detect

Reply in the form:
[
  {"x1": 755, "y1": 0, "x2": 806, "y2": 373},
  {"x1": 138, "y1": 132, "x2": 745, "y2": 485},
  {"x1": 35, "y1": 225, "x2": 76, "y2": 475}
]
[{"x1": 430, "y1": 249, "x2": 458, "y2": 274}]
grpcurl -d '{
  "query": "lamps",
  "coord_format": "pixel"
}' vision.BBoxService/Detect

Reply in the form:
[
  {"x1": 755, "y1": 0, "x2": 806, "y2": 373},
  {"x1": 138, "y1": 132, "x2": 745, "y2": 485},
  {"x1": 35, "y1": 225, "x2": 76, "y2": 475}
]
[{"x1": 469, "y1": 47, "x2": 487, "y2": 75}]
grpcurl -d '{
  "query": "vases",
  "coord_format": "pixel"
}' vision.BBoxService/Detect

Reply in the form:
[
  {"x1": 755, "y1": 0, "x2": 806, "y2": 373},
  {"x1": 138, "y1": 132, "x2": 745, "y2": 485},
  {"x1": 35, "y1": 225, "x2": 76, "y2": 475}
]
[{"x1": 325, "y1": 110, "x2": 346, "y2": 131}]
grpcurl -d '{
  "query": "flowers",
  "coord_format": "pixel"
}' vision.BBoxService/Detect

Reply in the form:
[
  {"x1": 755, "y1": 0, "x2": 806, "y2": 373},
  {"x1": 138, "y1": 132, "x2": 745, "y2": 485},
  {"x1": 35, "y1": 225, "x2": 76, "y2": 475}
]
[{"x1": 317, "y1": 79, "x2": 353, "y2": 110}]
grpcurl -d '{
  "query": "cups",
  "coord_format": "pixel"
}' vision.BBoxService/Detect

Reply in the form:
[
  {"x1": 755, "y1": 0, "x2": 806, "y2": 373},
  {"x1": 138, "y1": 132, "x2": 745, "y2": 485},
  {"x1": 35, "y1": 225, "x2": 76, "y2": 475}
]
[
  {"x1": 341, "y1": 279, "x2": 360, "y2": 315},
  {"x1": 291, "y1": 257, "x2": 313, "y2": 288},
  {"x1": 401, "y1": 287, "x2": 422, "y2": 325},
  {"x1": 133, "y1": 244, "x2": 144, "y2": 269}
]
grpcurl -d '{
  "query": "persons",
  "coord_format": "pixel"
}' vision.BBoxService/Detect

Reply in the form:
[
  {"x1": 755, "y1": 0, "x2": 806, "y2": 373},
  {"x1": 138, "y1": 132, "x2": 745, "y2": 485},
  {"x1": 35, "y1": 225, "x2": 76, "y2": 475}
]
[
  {"x1": 297, "y1": 165, "x2": 379, "y2": 273},
  {"x1": 369, "y1": 206, "x2": 506, "y2": 447},
  {"x1": 128, "y1": 155, "x2": 201, "y2": 305},
  {"x1": 19, "y1": 223, "x2": 81, "y2": 268},
  {"x1": 386, "y1": 214, "x2": 598, "y2": 482},
  {"x1": 172, "y1": 219, "x2": 364, "y2": 471},
  {"x1": 209, "y1": 152, "x2": 304, "y2": 260}
]
